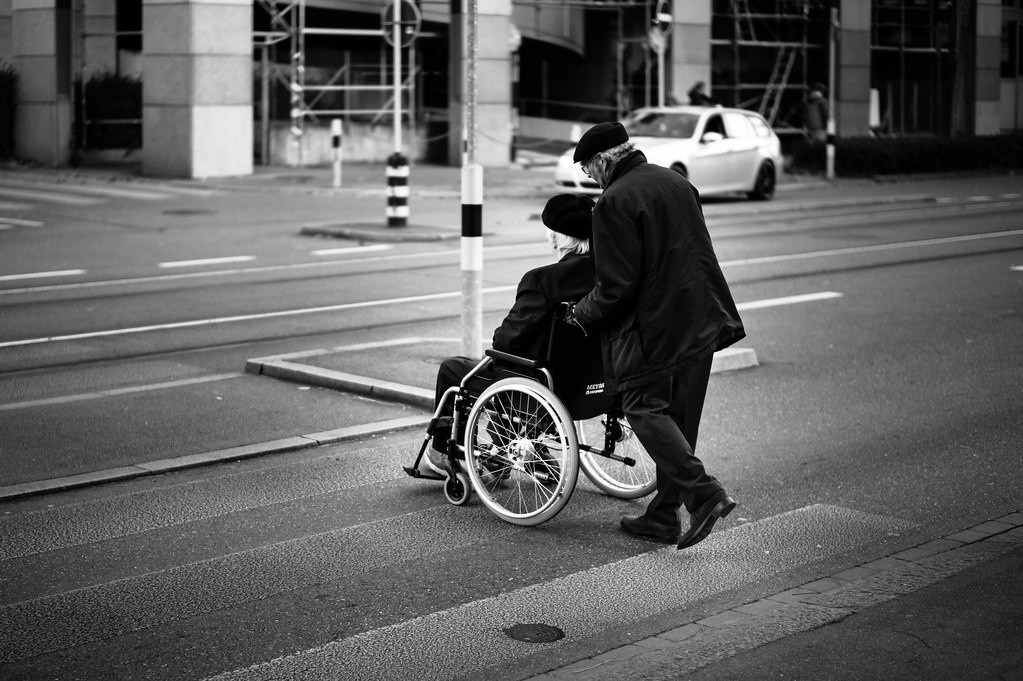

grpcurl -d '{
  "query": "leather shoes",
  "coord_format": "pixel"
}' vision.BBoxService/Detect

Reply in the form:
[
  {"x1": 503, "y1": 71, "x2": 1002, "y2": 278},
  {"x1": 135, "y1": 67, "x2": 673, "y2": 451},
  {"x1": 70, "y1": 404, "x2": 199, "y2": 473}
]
[
  {"x1": 677, "y1": 489, "x2": 739, "y2": 549},
  {"x1": 621, "y1": 514, "x2": 681, "y2": 545}
]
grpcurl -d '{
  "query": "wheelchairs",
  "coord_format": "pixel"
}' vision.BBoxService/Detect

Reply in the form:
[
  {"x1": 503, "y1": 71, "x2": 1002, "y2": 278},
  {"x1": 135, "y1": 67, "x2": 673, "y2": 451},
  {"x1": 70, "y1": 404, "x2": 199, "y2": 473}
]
[{"x1": 401, "y1": 299, "x2": 660, "y2": 527}]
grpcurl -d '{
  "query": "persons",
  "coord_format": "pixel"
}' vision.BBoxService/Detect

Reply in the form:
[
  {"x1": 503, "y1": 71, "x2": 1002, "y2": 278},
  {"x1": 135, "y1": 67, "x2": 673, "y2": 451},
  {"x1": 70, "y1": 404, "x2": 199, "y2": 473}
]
[
  {"x1": 568, "y1": 122, "x2": 748, "y2": 553},
  {"x1": 803, "y1": 86, "x2": 829, "y2": 174},
  {"x1": 689, "y1": 83, "x2": 713, "y2": 105},
  {"x1": 431, "y1": 195, "x2": 598, "y2": 477}
]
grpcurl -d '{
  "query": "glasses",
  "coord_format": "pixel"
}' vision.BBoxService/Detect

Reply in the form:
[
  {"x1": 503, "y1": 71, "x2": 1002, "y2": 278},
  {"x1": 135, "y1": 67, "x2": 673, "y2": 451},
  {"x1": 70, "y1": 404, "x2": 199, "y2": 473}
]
[{"x1": 582, "y1": 159, "x2": 594, "y2": 174}]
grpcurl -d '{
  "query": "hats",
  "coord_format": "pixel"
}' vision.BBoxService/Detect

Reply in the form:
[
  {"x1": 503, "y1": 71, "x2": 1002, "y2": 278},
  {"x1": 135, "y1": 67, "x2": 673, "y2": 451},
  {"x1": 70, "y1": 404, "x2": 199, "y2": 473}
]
[
  {"x1": 542, "y1": 193, "x2": 596, "y2": 239},
  {"x1": 573, "y1": 122, "x2": 629, "y2": 164}
]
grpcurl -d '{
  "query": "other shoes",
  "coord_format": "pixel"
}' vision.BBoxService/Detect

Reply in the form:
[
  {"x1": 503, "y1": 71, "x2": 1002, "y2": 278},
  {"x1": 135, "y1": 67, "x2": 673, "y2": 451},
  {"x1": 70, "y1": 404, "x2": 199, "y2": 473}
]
[
  {"x1": 425, "y1": 446, "x2": 475, "y2": 492},
  {"x1": 480, "y1": 458, "x2": 511, "y2": 484}
]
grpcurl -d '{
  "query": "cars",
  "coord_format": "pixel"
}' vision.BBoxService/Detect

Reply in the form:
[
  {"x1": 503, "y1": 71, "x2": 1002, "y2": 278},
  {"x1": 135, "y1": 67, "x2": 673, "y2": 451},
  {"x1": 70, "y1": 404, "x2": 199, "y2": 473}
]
[{"x1": 553, "y1": 103, "x2": 784, "y2": 205}]
